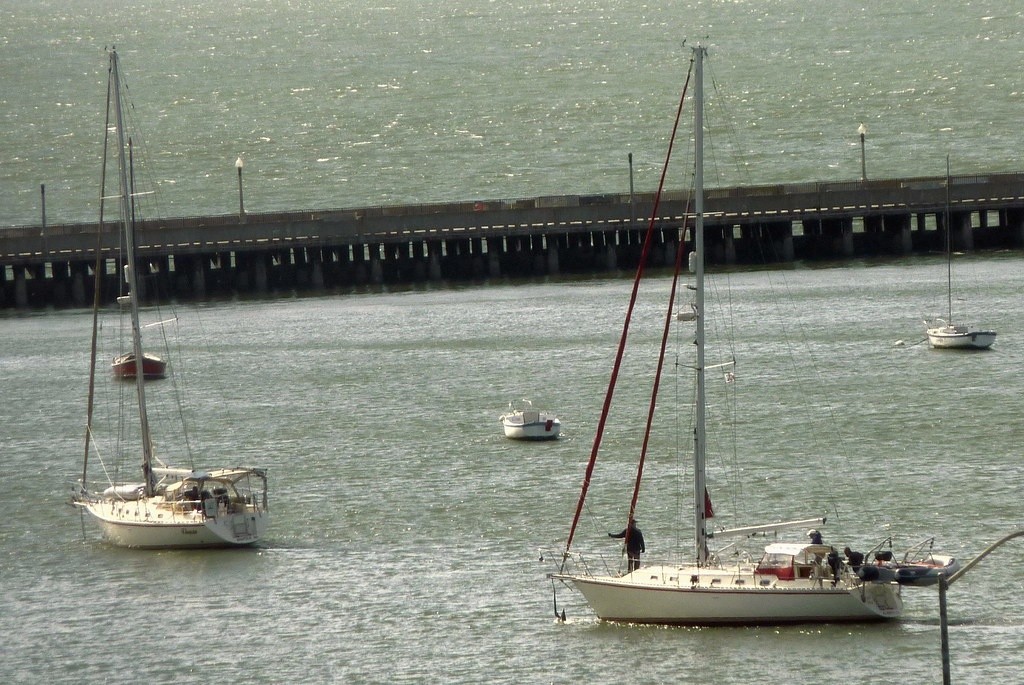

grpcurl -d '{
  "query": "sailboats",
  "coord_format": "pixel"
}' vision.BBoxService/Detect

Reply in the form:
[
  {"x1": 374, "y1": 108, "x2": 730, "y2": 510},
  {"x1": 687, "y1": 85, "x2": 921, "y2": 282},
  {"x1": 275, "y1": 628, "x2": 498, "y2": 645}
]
[
  {"x1": 540, "y1": 26, "x2": 957, "y2": 627},
  {"x1": 67, "y1": 47, "x2": 270, "y2": 549},
  {"x1": 100, "y1": 137, "x2": 166, "y2": 379},
  {"x1": 923, "y1": 154, "x2": 996, "y2": 351}
]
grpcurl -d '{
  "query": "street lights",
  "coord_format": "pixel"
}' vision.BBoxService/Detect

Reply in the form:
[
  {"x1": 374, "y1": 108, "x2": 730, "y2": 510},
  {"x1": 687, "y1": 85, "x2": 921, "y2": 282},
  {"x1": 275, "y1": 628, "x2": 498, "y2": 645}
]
[
  {"x1": 858, "y1": 123, "x2": 868, "y2": 183},
  {"x1": 235, "y1": 158, "x2": 247, "y2": 224}
]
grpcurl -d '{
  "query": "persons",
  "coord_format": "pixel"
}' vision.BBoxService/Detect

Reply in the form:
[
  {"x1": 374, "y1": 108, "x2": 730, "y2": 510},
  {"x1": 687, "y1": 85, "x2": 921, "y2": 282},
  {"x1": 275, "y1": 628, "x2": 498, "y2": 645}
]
[
  {"x1": 844, "y1": 547, "x2": 864, "y2": 572},
  {"x1": 609, "y1": 520, "x2": 645, "y2": 573},
  {"x1": 186, "y1": 486, "x2": 201, "y2": 509},
  {"x1": 827, "y1": 547, "x2": 845, "y2": 586},
  {"x1": 807, "y1": 529, "x2": 825, "y2": 564}
]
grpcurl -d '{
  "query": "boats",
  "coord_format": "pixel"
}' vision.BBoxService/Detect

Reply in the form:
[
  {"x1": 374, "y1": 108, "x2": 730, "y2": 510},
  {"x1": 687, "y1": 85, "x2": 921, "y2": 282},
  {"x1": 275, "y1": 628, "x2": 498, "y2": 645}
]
[
  {"x1": 829, "y1": 538, "x2": 959, "y2": 587},
  {"x1": 499, "y1": 399, "x2": 561, "y2": 439}
]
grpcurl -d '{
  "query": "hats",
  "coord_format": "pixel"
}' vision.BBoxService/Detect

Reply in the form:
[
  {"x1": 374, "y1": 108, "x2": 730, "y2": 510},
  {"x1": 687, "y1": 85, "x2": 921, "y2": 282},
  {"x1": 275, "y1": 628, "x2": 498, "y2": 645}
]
[{"x1": 631, "y1": 520, "x2": 638, "y2": 524}]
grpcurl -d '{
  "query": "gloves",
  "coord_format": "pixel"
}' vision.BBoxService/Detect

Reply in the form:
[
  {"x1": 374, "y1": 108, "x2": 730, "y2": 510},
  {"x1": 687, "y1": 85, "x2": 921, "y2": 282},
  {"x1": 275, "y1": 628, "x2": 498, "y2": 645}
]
[
  {"x1": 642, "y1": 550, "x2": 645, "y2": 553},
  {"x1": 608, "y1": 533, "x2": 611, "y2": 537}
]
